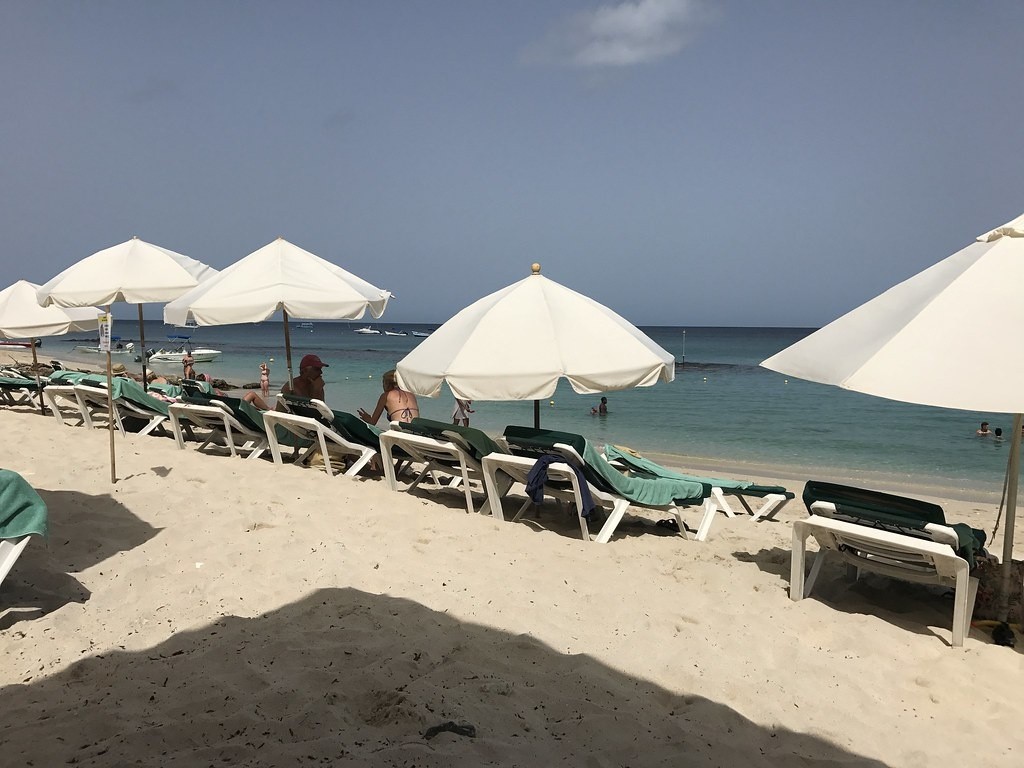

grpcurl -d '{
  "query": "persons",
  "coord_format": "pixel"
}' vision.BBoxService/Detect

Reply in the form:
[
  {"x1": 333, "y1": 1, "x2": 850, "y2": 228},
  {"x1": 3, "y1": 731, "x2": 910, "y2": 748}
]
[
  {"x1": 196, "y1": 373, "x2": 276, "y2": 411},
  {"x1": 451, "y1": 398, "x2": 475, "y2": 428},
  {"x1": 590, "y1": 397, "x2": 608, "y2": 416},
  {"x1": 182, "y1": 350, "x2": 195, "y2": 379},
  {"x1": 357, "y1": 370, "x2": 420, "y2": 476},
  {"x1": 259, "y1": 362, "x2": 270, "y2": 395},
  {"x1": 995, "y1": 428, "x2": 1002, "y2": 437},
  {"x1": 142, "y1": 369, "x2": 172, "y2": 385},
  {"x1": 977, "y1": 422, "x2": 992, "y2": 433},
  {"x1": 111, "y1": 363, "x2": 136, "y2": 382},
  {"x1": 275, "y1": 354, "x2": 330, "y2": 412}
]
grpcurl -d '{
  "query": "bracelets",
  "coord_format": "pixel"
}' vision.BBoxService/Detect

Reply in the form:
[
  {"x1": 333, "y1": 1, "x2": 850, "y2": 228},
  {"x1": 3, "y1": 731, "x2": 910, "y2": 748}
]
[{"x1": 309, "y1": 393, "x2": 321, "y2": 400}]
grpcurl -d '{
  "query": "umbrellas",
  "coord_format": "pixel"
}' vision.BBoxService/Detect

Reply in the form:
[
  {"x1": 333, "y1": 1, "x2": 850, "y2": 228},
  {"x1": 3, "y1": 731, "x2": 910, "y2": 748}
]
[
  {"x1": 0, "y1": 279, "x2": 113, "y2": 416},
  {"x1": 163, "y1": 236, "x2": 395, "y2": 395},
  {"x1": 36, "y1": 235, "x2": 219, "y2": 394},
  {"x1": 760, "y1": 212, "x2": 1024, "y2": 623},
  {"x1": 396, "y1": 263, "x2": 675, "y2": 429}
]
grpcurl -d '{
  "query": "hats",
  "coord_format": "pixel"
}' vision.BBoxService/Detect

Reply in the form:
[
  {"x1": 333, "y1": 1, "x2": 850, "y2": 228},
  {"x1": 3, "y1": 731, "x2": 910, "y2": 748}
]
[{"x1": 300, "y1": 355, "x2": 329, "y2": 368}]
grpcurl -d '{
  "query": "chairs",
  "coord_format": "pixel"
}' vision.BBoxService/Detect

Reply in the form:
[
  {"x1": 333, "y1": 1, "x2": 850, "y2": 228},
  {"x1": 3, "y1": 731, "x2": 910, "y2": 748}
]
[
  {"x1": 788, "y1": 480, "x2": 1000, "y2": 646},
  {"x1": 0, "y1": 371, "x2": 795, "y2": 583}
]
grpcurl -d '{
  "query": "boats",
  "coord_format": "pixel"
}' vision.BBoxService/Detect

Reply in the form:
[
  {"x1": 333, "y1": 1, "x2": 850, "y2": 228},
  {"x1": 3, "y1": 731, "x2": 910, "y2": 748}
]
[
  {"x1": 173, "y1": 320, "x2": 199, "y2": 329},
  {"x1": 384, "y1": 331, "x2": 407, "y2": 338},
  {"x1": 148, "y1": 335, "x2": 223, "y2": 363},
  {"x1": 296, "y1": 323, "x2": 313, "y2": 328},
  {"x1": 354, "y1": 326, "x2": 381, "y2": 334},
  {"x1": 413, "y1": 331, "x2": 430, "y2": 337},
  {"x1": 77, "y1": 337, "x2": 137, "y2": 354},
  {"x1": 0, "y1": 340, "x2": 35, "y2": 348}
]
